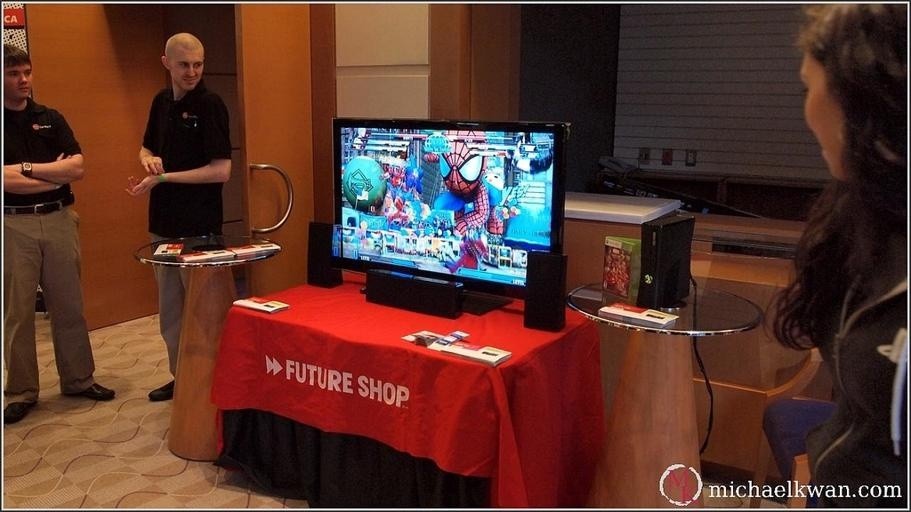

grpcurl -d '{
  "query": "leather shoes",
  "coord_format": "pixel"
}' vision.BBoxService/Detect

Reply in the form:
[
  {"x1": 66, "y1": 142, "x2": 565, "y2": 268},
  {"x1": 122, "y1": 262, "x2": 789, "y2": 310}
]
[
  {"x1": 148, "y1": 380, "x2": 176, "y2": 402},
  {"x1": 4, "y1": 397, "x2": 39, "y2": 425},
  {"x1": 62, "y1": 383, "x2": 116, "y2": 401}
]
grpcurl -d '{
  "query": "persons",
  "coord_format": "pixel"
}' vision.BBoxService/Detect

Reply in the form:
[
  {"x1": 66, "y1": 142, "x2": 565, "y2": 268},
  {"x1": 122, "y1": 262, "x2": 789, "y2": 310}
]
[
  {"x1": 764, "y1": 5, "x2": 908, "y2": 509},
  {"x1": 125, "y1": 32, "x2": 233, "y2": 402},
  {"x1": 3, "y1": 45, "x2": 117, "y2": 426}
]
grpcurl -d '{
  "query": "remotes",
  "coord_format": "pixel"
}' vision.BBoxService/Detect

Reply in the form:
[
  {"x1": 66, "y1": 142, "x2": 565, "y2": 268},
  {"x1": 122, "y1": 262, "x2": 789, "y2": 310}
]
[
  {"x1": 191, "y1": 239, "x2": 208, "y2": 250},
  {"x1": 208, "y1": 236, "x2": 225, "y2": 250}
]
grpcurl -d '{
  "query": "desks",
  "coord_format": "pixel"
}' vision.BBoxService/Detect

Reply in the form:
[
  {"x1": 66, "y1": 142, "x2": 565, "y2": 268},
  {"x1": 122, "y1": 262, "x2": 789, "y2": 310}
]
[
  {"x1": 575, "y1": 273, "x2": 766, "y2": 509},
  {"x1": 135, "y1": 230, "x2": 284, "y2": 457},
  {"x1": 226, "y1": 280, "x2": 605, "y2": 506}
]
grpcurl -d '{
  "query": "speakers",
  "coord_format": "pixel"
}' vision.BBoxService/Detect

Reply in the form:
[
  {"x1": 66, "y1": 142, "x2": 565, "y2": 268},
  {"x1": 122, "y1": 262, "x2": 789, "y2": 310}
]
[
  {"x1": 307, "y1": 222, "x2": 343, "y2": 288},
  {"x1": 524, "y1": 251, "x2": 568, "y2": 331},
  {"x1": 410, "y1": 275, "x2": 468, "y2": 319},
  {"x1": 366, "y1": 268, "x2": 414, "y2": 310}
]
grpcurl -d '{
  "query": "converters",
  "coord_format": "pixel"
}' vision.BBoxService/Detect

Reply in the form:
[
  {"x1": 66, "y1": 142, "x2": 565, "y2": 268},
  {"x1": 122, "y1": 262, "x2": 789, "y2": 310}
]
[{"x1": 569, "y1": 288, "x2": 601, "y2": 316}]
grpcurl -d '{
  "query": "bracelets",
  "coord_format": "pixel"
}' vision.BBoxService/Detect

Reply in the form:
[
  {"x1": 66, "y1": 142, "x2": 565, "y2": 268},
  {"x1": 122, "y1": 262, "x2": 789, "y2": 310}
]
[{"x1": 157, "y1": 169, "x2": 169, "y2": 187}]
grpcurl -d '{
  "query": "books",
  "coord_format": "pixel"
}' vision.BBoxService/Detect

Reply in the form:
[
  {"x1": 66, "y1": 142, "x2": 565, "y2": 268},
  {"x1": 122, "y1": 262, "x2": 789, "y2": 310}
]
[
  {"x1": 234, "y1": 295, "x2": 291, "y2": 315},
  {"x1": 598, "y1": 302, "x2": 680, "y2": 329},
  {"x1": 442, "y1": 339, "x2": 513, "y2": 368},
  {"x1": 150, "y1": 241, "x2": 282, "y2": 265}
]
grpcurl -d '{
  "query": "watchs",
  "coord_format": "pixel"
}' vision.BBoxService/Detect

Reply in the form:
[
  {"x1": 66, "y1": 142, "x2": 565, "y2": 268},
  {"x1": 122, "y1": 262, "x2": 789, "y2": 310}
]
[{"x1": 18, "y1": 160, "x2": 35, "y2": 178}]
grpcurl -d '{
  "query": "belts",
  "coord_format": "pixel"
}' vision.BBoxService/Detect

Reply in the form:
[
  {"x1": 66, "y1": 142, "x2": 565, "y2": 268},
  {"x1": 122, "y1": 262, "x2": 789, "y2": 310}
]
[{"x1": 5, "y1": 193, "x2": 76, "y2": 215}]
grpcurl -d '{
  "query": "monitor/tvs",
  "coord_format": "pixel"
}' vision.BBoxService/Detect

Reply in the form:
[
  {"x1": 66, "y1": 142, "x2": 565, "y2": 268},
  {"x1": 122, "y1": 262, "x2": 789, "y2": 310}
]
[{"x1": 331, "y1": 115, "x2": 571, "y2": 316}]
